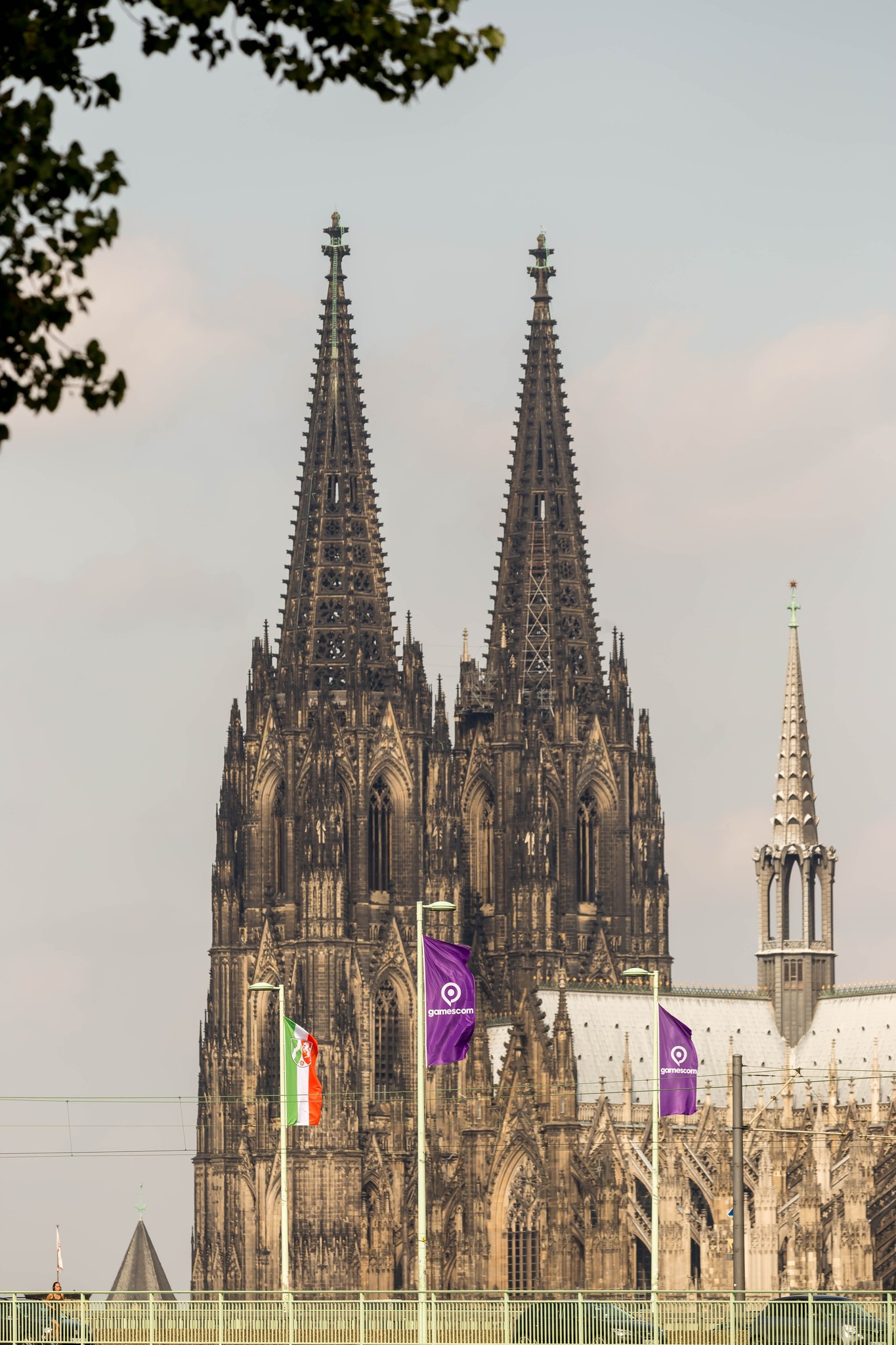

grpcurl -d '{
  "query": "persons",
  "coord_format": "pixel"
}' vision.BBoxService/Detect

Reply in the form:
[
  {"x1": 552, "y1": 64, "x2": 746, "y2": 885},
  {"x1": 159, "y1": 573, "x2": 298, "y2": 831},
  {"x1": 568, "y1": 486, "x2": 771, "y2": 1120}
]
[{"x1": 40, "y1": 1282, "x2": 64, "y2": 1345}]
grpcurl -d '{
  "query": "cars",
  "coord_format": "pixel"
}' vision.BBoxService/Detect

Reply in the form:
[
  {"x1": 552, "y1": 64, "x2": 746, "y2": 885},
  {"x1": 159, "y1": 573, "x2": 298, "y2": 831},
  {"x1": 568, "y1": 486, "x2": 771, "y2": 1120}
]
[
  {"x1": 0, "y1": 1297, "x2": 94, "y2": 1345},
  {"x1": 749, "y1": 1295, "x2": 894, "y2": 1345},
  {"x1": 512, "y1": 1299, "x2": 668, "y2": 1345}
]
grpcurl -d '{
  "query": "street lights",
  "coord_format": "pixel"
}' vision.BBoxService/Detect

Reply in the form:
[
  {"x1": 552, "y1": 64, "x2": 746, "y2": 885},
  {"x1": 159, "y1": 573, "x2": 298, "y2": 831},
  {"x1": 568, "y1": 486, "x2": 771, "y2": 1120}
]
[
  {"x1": 247, "y1": 980, "x2": 291, "y2": 1314},
  {"x1": 416, "y1": 898, "x2": 459, "y2": 1345},
  {"x1": 621, "y1": 967, "x2": 659, "y2": 1344}
]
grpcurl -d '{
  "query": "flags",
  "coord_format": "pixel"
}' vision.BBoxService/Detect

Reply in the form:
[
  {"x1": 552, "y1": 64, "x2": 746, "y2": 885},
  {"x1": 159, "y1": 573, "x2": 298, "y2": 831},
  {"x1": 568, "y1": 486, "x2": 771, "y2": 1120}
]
[
  {"x1": 422, "y1": 933, "x2": 476, "y2": 1066},
  {"x1": 283, "y1": 1014, "x2": 323, "y2": 1126},
  {"x1": 56, "y1": 1227, "x2": 63, "y2": 1273},
  {"x1": 657, "y1": 1003, "x2": 698, "y2": 1117}
]
